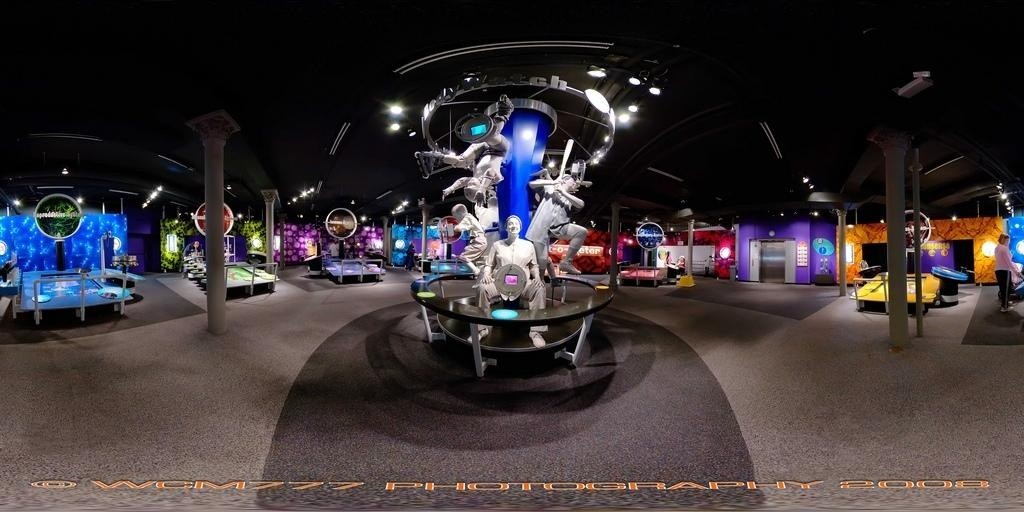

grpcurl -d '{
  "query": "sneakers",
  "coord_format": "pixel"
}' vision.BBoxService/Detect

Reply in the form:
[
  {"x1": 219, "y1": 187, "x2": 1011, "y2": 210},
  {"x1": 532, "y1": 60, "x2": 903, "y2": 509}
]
[{"x1": 1000, "y1": 300, "x2": 1017, "y2": 312}]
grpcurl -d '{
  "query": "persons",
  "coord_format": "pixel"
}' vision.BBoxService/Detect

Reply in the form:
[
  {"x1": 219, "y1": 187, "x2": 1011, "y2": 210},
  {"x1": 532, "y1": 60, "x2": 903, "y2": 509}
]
[
  {"x1": 328, "y1": 238, "x2": 340, "y2": 259},
  {"x1": 679, "y1": 256, "x2": 685, "y2": 272},
  {"x1": 180, "y1": 241, "x2": 201, "y2": 278},
  {"x1": 995, "y1": 234, "x2": 1022, "y2": 312},
  {"x1": 705, "y1": 256, "x2": 712, "y2": 278},
  {"x1": 422, "y1": 91, "x2": 588, "y2": 347},
  {"x1": 405, "y1": 242, "x2": 416, "y2": 272}
]
